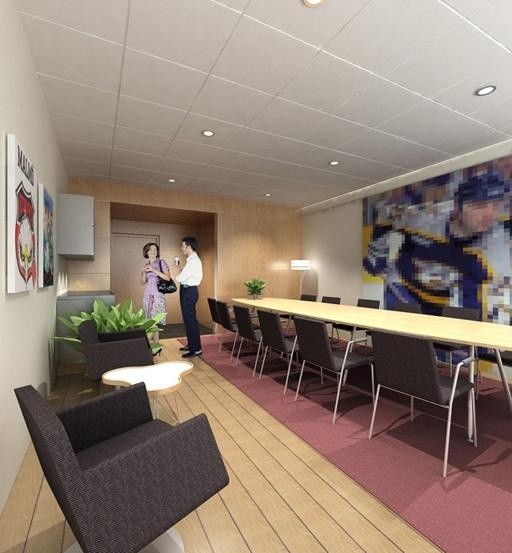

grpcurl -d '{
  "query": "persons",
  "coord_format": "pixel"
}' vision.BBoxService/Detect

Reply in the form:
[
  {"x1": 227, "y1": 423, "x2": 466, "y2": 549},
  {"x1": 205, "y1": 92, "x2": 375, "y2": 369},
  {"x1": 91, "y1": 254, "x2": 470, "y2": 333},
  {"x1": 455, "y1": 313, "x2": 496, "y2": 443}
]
[
  {"x1": 141, "y1": 243, "x2": 171, "y2": 356},
  {"x1": 362, "y1": 169, "x2": 512, "y2": 374},
  {"x1": 175, "y1": 237, "x2": 203, "y2": 358}
]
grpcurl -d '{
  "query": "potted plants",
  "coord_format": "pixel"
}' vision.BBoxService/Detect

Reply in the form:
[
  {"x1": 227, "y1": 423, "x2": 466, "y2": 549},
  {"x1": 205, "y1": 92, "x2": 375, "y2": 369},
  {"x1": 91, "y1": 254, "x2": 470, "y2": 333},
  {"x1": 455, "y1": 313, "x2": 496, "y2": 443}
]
[
  {"x1": 50, "y1": 298, "x2": 169, "y2": 380},
  {"x1": 246, "y1": 279, "x2": 267, "y2": 301}
]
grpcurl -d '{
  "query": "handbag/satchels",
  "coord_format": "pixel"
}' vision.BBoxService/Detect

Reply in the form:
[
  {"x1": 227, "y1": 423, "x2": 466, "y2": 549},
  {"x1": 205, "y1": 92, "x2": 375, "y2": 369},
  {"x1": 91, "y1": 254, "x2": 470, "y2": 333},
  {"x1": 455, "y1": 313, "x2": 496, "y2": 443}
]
[{"x1": 156, "y1": 259, "x2": 177, "y2": 294}]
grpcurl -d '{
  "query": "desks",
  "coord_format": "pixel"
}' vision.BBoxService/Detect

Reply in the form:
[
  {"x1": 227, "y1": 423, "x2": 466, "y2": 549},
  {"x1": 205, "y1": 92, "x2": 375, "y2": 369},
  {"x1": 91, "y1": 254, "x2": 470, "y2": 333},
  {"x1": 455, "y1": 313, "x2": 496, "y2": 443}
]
[{"x1": 231, "y1": 295, "x2": 512, "y2": 444}]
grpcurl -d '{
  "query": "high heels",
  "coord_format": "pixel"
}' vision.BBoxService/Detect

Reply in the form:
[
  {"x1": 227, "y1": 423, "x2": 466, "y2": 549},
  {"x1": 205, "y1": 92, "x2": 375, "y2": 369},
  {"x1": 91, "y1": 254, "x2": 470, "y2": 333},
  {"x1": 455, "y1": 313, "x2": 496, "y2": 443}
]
[{"x1": 153, "y1": 347, "x2": 162, "y2": 357}]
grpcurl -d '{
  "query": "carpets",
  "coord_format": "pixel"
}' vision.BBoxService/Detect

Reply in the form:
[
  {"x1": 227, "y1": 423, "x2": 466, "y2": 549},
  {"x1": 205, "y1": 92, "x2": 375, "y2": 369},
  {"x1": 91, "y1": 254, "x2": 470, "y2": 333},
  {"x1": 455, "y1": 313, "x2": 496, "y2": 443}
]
[{"x1": 175, "y1": 326, "x2": 512, "y2": 553}]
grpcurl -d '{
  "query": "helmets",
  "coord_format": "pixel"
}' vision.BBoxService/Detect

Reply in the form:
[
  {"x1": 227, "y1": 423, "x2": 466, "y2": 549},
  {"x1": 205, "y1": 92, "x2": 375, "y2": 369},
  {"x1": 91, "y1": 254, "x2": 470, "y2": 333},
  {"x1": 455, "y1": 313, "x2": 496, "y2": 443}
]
[{"x1": 457, "y1": 168, "x2": 505, "y2": 211}]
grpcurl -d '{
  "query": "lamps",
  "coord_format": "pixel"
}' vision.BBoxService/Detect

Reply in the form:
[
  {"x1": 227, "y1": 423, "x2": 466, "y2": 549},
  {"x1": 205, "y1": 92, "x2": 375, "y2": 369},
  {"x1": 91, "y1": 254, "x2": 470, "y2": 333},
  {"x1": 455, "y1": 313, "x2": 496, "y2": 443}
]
[{"x1": 291, "y1": 259, "x2": 311, "y2": 300}]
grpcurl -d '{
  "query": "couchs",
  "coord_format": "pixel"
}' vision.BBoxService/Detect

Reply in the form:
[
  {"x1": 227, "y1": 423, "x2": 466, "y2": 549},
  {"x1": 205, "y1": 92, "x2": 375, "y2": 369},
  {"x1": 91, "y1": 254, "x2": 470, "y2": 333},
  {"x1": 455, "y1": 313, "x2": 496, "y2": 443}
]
[
  {"x1": 14, "y1": 379, "x2": 230, "y2": 552},
  {"x1": 78, "y1": 319, "x2": 155, "y2": 381}
]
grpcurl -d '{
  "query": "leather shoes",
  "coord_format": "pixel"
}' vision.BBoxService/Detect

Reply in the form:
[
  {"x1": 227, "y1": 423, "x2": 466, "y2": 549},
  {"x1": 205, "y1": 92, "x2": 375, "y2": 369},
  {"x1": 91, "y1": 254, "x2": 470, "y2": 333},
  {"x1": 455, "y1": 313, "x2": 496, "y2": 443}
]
[
  {"x1": 179, "y1": 344, "x2": 201, "y2": 350},
  {"x1": 182, "y1": 349, "x2": 202, "y2": 358}
]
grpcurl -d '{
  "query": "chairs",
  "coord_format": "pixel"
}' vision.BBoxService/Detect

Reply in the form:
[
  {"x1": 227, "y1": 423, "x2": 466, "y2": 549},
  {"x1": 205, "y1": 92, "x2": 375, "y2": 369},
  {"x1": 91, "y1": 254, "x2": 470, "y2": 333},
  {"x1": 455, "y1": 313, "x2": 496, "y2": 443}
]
[
  {"x1": 257, "y1": 310, "x2": 301, "y2": 381},
  {"x1": 215, "y1": 301, "x2": 260, "y2": 352},
  {"x1": 333, "y1": 299, "x2": 379, "y2": 353},
  {"x1": 300, "y1": 294, "x2": 315, "y2": 302},
  {"x1": 320, "y1": 295, "x2": 342, "y2": 344},
  {"x1": 232, "y1": 306, "x2": 264, "y2": 366},
  {"x1": 292, "y1": 316, "x2": 376, "y2": 427},
  {"x1": 207, "y1": 298, "x2": 217, "y2": 333},
  {"x1": 367, "y1": 329, "x2": 479, "y2": 479}
]
